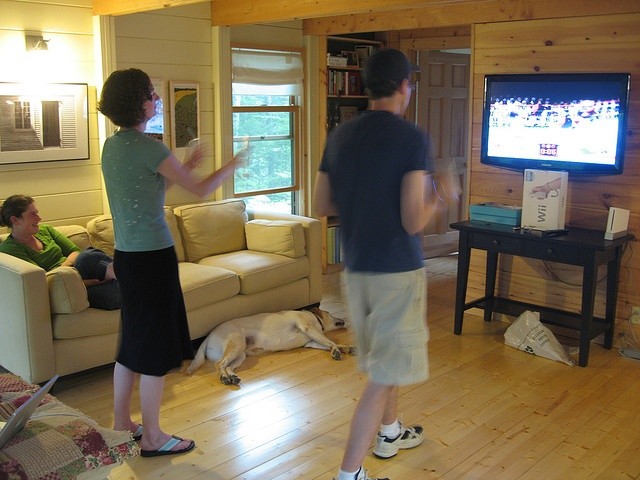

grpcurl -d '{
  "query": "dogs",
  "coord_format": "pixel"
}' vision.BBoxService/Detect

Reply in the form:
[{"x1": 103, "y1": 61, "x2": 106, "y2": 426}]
[{"x1": 185, "y1": 306, "x2": 358, "y2": 385}]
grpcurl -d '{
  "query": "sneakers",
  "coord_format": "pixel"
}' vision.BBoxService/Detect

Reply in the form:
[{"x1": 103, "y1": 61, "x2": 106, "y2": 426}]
[
  {"x1": 374, "y1": 420, "x2": 425, "y2": 459},
  {"x1": 332, "y1": 465, "x2": 391, "y2": 480}
]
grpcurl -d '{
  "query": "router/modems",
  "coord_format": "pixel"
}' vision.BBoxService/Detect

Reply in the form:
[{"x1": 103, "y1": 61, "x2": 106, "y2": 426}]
[{"x1": 604, "y1": 206, "x2": 631, "y2": 241}]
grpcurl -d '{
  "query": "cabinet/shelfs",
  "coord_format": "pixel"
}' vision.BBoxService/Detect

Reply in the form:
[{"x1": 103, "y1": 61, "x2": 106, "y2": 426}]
[
  {"x1": 317, "y1": 36, "x2": 384, "y2": 275},
  {"x1": 446, "y1": 219, "x2": 633, "y2": 368}
]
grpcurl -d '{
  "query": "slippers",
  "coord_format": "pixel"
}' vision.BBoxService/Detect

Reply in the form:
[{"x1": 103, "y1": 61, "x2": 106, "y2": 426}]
[
  {"x1": 140, "y1": 435, "x2": 195, "y2": 458},
  {"x1": 113, "y1": 423, "x2": 144, "y2": 440}
]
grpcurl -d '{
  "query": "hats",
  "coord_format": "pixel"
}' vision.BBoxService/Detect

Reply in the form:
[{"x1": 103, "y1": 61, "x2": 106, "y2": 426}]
[{"x1": 362, "y1": 47, "x2": 420, "y2": 83}]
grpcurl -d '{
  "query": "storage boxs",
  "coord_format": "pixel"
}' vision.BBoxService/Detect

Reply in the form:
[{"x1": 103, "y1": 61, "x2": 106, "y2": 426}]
[{"x1": 468, "y1": 201, "x2": 523, "y2": 227}]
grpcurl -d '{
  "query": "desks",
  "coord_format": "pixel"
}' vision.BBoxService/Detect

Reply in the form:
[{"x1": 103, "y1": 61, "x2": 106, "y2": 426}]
[{"x1": 0, "y1": 364, "x2": 142, "y2": 480}]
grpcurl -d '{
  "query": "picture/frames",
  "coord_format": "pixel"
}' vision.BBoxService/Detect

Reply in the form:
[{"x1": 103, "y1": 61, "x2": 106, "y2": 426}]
[
  {"x1": 169, "y1": 80, "x2": 201, "y2": 169},
  {"x1": 0, "y1": 81, "x2": 90, "y2": 166}
]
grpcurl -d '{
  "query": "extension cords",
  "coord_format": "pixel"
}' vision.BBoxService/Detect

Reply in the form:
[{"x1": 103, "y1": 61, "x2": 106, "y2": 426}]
[{"x1": 620, "y1": 347, "x2": 639, "y2": 361}]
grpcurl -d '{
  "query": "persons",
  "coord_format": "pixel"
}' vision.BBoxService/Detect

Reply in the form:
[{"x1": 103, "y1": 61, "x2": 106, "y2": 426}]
[
  {"x1": 313, "y1": 49, "x2": 461, "y2": 480},
  {"x1": 95, "y1": 68, "x2": 253, "y2": 458},
  {"x1": 0, "y1": 193, "x2": 122, "y2": 310}
]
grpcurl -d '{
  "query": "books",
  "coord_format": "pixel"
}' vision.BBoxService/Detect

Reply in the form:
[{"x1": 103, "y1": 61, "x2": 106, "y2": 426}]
[
  {"x1": 327, "y1": 45, "x2": 377, "y2": 68},
  {"x1": 322, "y1": 227, "x2": 353, "y2": 263},
  {"x1": 327, "y1": 70, "x2": 362, "y2": 96},
  {"x1": 339, "y1": 106, "x2": 358, "y2": 123}
]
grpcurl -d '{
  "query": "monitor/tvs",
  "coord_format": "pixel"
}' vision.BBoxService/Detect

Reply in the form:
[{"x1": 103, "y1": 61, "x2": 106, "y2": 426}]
[{"x1": 480, "y1": 72, "x2": 631, "y2": 175}]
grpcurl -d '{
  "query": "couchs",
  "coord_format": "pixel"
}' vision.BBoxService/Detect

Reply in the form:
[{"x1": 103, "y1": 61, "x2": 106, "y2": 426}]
[{"x1": 0, "y1": 196, "x2": 326, "y2": 393}]
